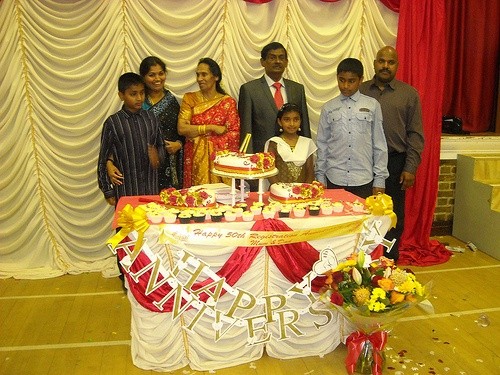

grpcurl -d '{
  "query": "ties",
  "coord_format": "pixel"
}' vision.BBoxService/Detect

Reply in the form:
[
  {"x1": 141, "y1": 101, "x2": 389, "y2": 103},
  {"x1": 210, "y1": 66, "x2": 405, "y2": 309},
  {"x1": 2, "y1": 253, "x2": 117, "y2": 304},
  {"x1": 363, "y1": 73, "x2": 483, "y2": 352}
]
[{"x1": 273, "y1": 83, "x2": 284, "y2": 110}]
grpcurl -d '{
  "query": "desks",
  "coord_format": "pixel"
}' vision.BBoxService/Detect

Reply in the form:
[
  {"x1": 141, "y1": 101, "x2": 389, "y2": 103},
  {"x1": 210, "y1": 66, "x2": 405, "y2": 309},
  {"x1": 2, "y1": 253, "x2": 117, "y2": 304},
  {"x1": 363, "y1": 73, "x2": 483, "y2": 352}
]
[{"x1": 112, "y1": 188, "x2": 397, "y2": 371}]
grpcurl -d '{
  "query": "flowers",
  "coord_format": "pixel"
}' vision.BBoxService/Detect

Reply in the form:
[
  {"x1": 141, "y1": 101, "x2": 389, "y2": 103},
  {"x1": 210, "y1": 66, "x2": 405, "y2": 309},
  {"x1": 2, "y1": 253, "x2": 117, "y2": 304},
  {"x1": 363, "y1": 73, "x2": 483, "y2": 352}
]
[
  {"x1": 311, "y1": 249, "x2": 427, "y2": 375},
  {"x1": 250, "y1": 152, "x2": 275, "y2": 170},
  {"x1": 160, "y1": 187, "x2": 208, "y2": 207},
  {"x1": 293, "y1": 180, "x2": 323, "y2": 198}
]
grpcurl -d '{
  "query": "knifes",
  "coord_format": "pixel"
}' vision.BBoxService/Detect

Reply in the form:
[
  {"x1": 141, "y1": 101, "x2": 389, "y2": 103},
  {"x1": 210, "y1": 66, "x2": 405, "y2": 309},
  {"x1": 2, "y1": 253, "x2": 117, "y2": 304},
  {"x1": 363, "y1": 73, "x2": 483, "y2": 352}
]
[{"x1": 138, "y1": 197, "x2": 165, "y2": 205}]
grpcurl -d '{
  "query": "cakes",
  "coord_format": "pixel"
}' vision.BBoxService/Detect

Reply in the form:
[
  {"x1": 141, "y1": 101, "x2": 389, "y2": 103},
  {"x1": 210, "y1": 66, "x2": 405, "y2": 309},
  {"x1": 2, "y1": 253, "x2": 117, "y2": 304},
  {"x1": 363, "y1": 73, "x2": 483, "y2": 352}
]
[
  {"x1": 213, "y1": 133, "x2": 275, "y2": 174},
  {"x1": 160, "y1": 187, "x2": 216, "y2": 207},
  {"x1": 270, "y1": 180, "x2": 324, "y2": 203}
]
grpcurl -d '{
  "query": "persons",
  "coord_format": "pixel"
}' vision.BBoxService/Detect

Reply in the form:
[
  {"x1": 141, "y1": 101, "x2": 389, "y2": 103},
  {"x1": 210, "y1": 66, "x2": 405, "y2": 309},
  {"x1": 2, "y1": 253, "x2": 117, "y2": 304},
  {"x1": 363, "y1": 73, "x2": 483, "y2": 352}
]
[
  {"x1": 359, "y1": 45, "x2": 425, "y2": 265},
  {"x1": 106, "y1": 56, "x2": 185, "y2": 195},
  {"x1": 97, "y1": 72, "x2": 166, "y2": 296},
  {"x1": 177, "y1": 58, "x2": 239, "y2": 189},
  {"x1": 314, "y1": 58, "x2": 390, "y2": 199},
  {"x1": 237, "y1": 42, "x2": 313, "y2": 192},
  {"x1": 263, "y1": 103, "x2": 317, "y2": 190}
]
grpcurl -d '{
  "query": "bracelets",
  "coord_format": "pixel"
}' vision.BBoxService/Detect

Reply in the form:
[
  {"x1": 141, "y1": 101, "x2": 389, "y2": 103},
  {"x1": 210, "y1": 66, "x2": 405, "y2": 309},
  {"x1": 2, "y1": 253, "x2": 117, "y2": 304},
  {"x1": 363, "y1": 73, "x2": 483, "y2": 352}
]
[
  {"x1": 107, "y1": 157, "x2": 115, "y2": 164},
  {"x1": 198, "y1": 125, "x2": 206, "y2": 136}
]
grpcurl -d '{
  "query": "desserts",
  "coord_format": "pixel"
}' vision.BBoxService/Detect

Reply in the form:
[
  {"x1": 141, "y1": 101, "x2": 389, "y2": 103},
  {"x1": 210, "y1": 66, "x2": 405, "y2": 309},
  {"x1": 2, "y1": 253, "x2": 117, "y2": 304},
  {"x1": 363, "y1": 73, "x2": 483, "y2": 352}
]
[{"x1": 145, "y1": 201, "x2": 364, "y2": 224}]
